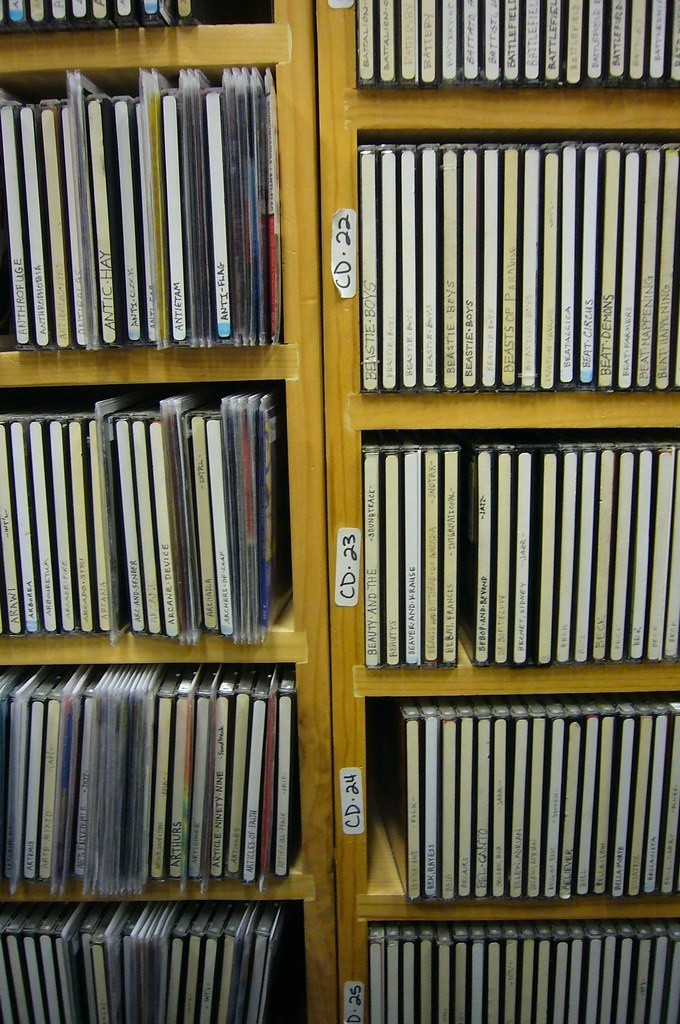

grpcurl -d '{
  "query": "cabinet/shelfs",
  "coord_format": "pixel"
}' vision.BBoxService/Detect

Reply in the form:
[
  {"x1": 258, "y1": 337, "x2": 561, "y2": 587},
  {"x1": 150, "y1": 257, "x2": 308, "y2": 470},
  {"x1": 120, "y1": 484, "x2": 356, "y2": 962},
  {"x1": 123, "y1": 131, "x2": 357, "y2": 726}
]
[
  {"x1": 316, "y1": 0, "x2": 679, "y2": 1024},
  {"x1": 1, "y1": 1, "x2": 340, "y2": 1024}
]
